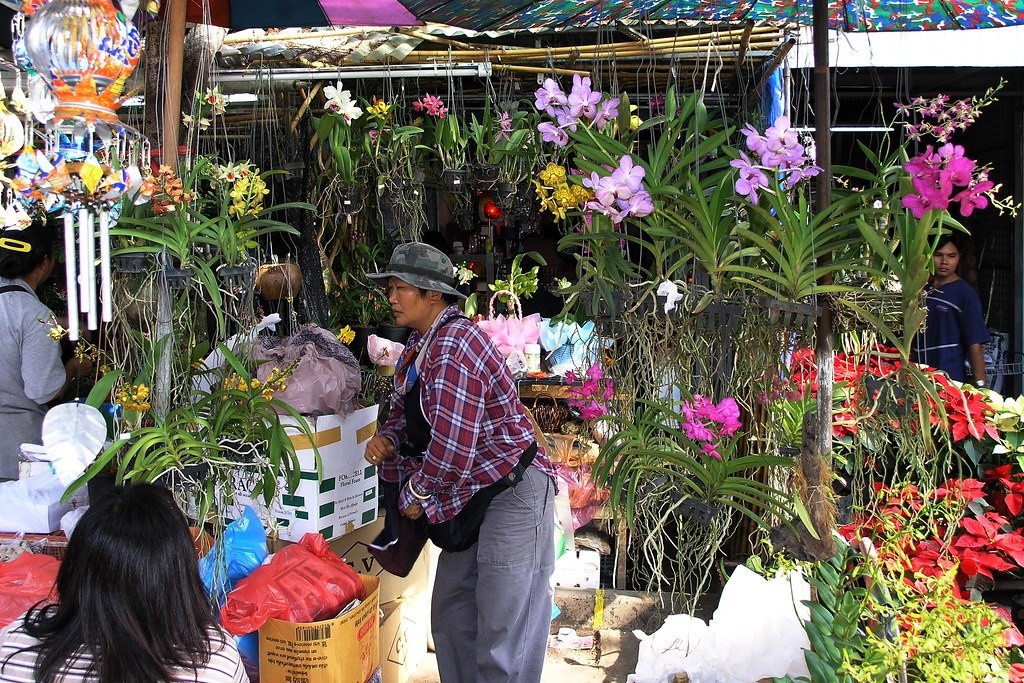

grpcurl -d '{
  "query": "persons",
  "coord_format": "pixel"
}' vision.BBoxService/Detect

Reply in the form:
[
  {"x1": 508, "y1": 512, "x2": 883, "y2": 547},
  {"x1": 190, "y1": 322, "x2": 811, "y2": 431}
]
[
  {"x1": 905, "y1": 232, "x2": 986, "y2": 393},
  {"x1": 0, "y1": 219, "x2": 94, "y2": 484},
  {"x1": 362, "y1": 240, "x2": 561, "y2": 683},
  {"x1": 0, "y1": 480, "x2": 253, "y2": 683}
]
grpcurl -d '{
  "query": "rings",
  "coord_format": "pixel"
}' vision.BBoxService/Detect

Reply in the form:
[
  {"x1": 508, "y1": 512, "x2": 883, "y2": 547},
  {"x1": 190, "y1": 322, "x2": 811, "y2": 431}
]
[{"x1": 372, "y1": 455, "x2": 376, "y2": 461}]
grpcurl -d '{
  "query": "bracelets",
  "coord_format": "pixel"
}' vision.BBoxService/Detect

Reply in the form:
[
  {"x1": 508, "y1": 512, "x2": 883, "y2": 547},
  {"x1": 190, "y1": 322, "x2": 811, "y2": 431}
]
[
  {"x1": 408, "y1": 476, "x2": 431, "y2": 500},
  {"x1": 66, "y1": 374, "x2": 77, "y2": 383}
]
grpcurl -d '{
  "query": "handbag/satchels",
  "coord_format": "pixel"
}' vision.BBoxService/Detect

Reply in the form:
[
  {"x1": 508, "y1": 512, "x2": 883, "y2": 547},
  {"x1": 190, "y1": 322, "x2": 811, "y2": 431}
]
[{"x1": 424, "y1": 441, "x2": 537, "y2": 553}]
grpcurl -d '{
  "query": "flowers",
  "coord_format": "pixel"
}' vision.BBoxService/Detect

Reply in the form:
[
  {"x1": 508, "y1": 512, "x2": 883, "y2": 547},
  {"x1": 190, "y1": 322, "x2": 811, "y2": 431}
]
[{"x1": 38, "y1": 0, "x2": 1024, "y2": 565}]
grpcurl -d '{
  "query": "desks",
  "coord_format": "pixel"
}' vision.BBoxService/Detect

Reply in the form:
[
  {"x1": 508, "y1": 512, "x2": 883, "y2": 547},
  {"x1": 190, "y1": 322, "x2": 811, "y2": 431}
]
[{"x1": 515, "y1": 378, "x2": 634, "y2": 440}]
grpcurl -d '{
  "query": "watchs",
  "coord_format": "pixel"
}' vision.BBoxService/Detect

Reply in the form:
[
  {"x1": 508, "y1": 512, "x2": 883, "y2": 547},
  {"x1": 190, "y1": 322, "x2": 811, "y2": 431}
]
[{"x1": 974, "y1": 378, "x2": 986, "y2": 387}]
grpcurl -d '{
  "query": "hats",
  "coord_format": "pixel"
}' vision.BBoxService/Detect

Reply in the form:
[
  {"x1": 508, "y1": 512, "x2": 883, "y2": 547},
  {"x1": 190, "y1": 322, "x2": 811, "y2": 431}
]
[{"x1": 365, "y1": 242, "x2": 468, "y2": 299}]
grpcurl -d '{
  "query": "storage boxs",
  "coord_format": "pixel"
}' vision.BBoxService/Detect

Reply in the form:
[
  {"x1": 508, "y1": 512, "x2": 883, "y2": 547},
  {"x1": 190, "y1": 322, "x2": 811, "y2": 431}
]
[
  {"x1": 268, "y1": 515, "x2": 431, "y2": 606},
  {"x1": 378, "y1": 587, "x2": 428, "y2": 683},
  {"x1": 260, "y1": 573, "x2": 381, "y2": 683},
  {"x1": 191, "y1": 402, "x2": 380, "y2": 541}
]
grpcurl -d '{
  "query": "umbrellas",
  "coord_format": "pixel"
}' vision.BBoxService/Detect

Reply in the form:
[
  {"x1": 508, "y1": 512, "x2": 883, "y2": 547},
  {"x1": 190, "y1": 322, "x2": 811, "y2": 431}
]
[
  {"x1": 395, "y1": 0, "x2": 1024, "y2": 486},
  {"x1": 0, "y1": 0, "x2": 426, "y2": 430}
]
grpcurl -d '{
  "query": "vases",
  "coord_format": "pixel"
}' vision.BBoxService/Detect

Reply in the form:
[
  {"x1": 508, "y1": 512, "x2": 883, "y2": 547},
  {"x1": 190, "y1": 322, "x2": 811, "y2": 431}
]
[
  {"x1": 582, "y1": 291, "x2": 596, "y2": 316},
  {"x1": 606, "y1": 292, "x2": 628, "y2": 317},
  {"x1": 341, "y1": 194, "x2": 361, "y2": 212},
  {"x1": 641, "y1": 295, "x2": 686, "y2": 321},
  {"x1": 161, "y1": 269, "x2": 195, "y2": 290},
  {"x1": 220, "y1": 266, "x2": 256, "y2": 294},
  {"x1": 444, "y1": 169, "x2": 468, "y2": 195},
  {"x1": 499, "y1": 181, "x2": 516, "y2": 197},
  {"x1": 672, "y1": 494, "x2": 718, "y2": 525},
  {"x1": 114, "y1": 253, "x2": 149, "y2": 273},
  {"x1": 474, "y1": 165, "x2": 496, "y2": 180},
  {"x1": 390, "y1": 177, "x2": 406, "y2": 192}
]
